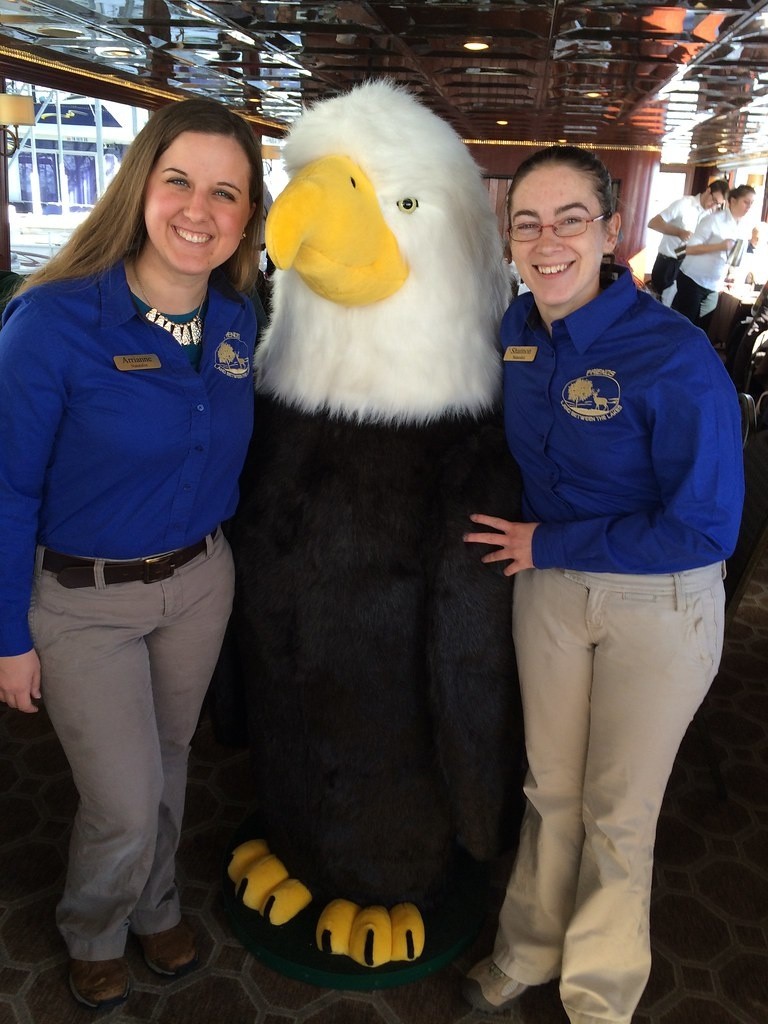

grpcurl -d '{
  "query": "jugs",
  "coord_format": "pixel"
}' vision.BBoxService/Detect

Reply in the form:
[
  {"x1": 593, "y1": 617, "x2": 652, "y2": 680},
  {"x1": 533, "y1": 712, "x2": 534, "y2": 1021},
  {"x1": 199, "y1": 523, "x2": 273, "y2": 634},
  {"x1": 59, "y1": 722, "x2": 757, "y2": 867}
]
[{"x1": 725, "y1": 238, "x2": 746, "y2": 267}]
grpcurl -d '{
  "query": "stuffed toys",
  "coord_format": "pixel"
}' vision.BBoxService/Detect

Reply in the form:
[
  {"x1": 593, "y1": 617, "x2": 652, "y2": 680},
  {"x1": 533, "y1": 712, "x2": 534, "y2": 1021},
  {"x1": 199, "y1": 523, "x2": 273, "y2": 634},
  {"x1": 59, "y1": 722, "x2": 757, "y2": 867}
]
[{"x1": 204, "y1": 80, "x2": 517, "y2": 966}]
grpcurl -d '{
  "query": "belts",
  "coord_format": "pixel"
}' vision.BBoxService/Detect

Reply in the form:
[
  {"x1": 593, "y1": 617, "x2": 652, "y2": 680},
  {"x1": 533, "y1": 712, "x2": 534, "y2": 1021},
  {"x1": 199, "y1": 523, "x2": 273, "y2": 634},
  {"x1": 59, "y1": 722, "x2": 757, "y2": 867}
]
[{"x1": 33, "y1": 525, "x2": 219, "y2": 591}]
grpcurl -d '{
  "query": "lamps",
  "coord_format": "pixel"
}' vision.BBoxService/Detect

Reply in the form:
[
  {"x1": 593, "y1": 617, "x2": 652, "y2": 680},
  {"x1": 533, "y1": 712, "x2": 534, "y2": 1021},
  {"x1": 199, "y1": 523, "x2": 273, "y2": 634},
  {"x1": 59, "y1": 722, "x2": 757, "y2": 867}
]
[{"x1": 0, "y1": 93, "x2": 36, "y2": 158}]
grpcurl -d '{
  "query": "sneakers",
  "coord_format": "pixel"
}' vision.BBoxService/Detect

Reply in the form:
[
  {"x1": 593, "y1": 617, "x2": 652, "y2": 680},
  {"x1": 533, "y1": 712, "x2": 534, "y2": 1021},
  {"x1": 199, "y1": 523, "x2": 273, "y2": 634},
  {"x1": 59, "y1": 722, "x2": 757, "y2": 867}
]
[
  {"x1": 140, "y1": 920, "x2": 200, "y2": 975},
  {"x1": 459, "y1": 955, "x2": 529, "y2": 1012},
  {"x1": 68, "y1": 957, "x2": 130, "y2": 1011}
]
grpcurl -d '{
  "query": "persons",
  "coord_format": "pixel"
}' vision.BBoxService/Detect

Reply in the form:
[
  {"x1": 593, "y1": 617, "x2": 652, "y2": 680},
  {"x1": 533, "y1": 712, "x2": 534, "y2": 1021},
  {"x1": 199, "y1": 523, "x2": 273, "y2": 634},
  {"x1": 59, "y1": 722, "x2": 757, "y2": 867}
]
[
  {"x1": 1, "y1": 100, "x2": 260, "y2": 1006},
  {"x1": 647, "y1": 179, "x2": 768, "y2": 428},
  {"x1": 463, "y1": 146, "x2": 746, "y2": 1023}
]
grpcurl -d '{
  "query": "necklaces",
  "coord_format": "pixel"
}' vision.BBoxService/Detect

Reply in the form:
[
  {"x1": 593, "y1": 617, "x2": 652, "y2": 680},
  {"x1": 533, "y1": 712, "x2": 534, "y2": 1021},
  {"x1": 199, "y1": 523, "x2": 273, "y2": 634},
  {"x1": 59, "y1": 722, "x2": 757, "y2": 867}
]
[{"x1": 132, "y1": 259, "x2": 207, "y2": 345}]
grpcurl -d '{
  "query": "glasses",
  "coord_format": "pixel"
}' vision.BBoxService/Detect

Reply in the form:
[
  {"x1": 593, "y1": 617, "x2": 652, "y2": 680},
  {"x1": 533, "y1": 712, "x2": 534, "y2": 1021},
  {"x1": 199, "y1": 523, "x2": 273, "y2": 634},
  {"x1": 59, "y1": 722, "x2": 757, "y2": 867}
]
[
  {"x1": 506, "y1": 210, "x2": 614, "y2": 243},
  {"x1": 711, "y1": 191, "x2": 723, "y2": 206}
]
